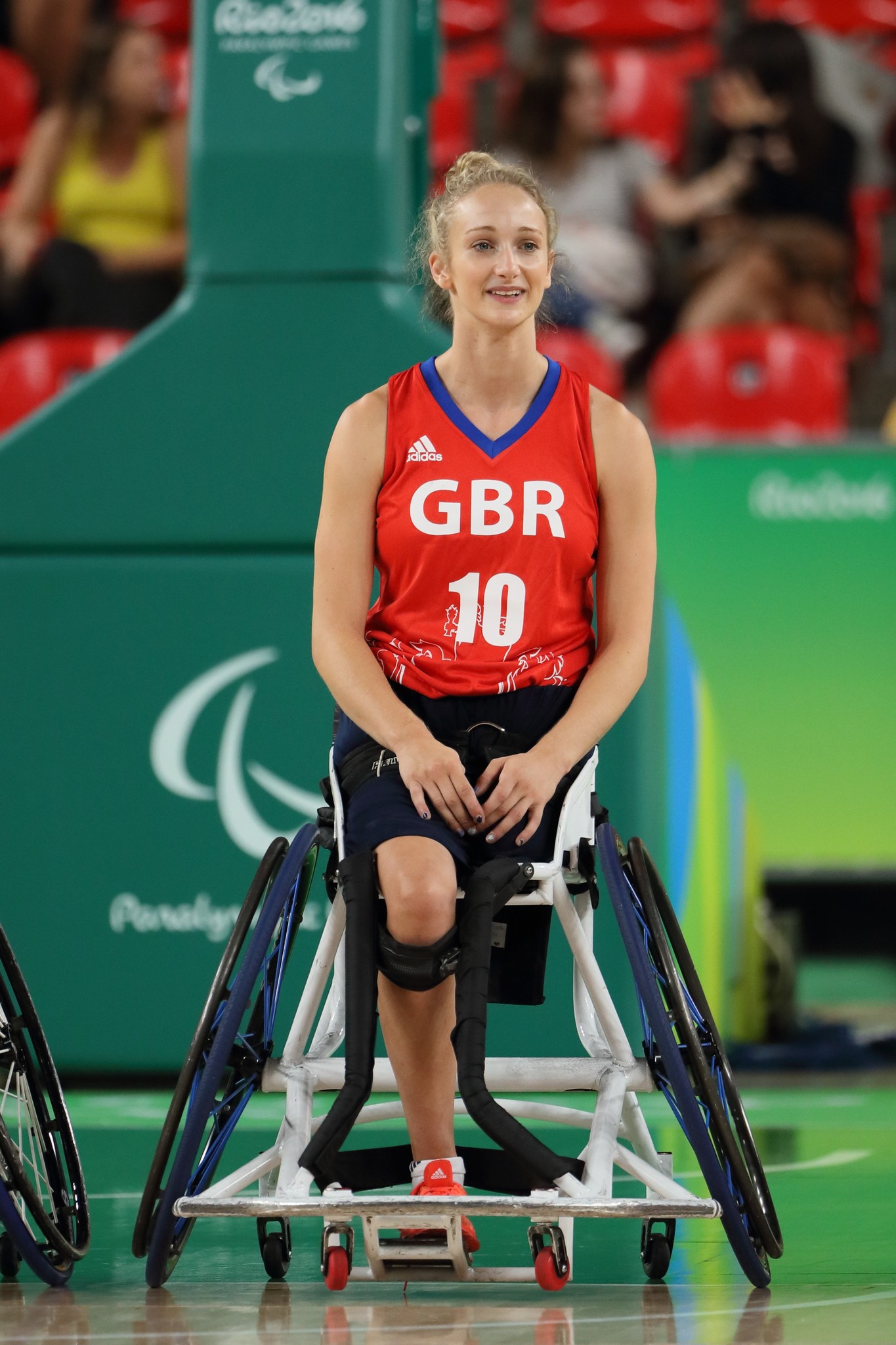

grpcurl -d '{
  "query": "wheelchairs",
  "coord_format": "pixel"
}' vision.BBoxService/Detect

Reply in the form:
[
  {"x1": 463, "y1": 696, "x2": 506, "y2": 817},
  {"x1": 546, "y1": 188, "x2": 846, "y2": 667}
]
[
  {"x1": 130, "y1": 768, "x2": 784, "y2": 1288},
  {"x1": 0, "y1": 927, "x2": 90, "y2": 1288}
]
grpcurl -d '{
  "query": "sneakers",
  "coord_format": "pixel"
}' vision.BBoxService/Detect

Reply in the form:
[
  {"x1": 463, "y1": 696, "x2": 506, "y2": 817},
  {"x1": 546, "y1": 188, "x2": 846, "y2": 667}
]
[{"x1": 395, "y1": 1159, "x2": 480, "y2": 1253}]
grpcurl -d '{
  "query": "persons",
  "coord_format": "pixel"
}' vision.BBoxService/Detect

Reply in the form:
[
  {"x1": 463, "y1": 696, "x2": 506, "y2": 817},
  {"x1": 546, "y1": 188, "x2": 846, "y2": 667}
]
[
  {"x1": 468, "y1": 0, "x2": 896, "y2": 442},
  {"x1": 0, "y1": 0, "x2": 185, "y2": 345},
  {"x1": 310, "y1": 152, "x2": 657, "y2": 1252}
]
